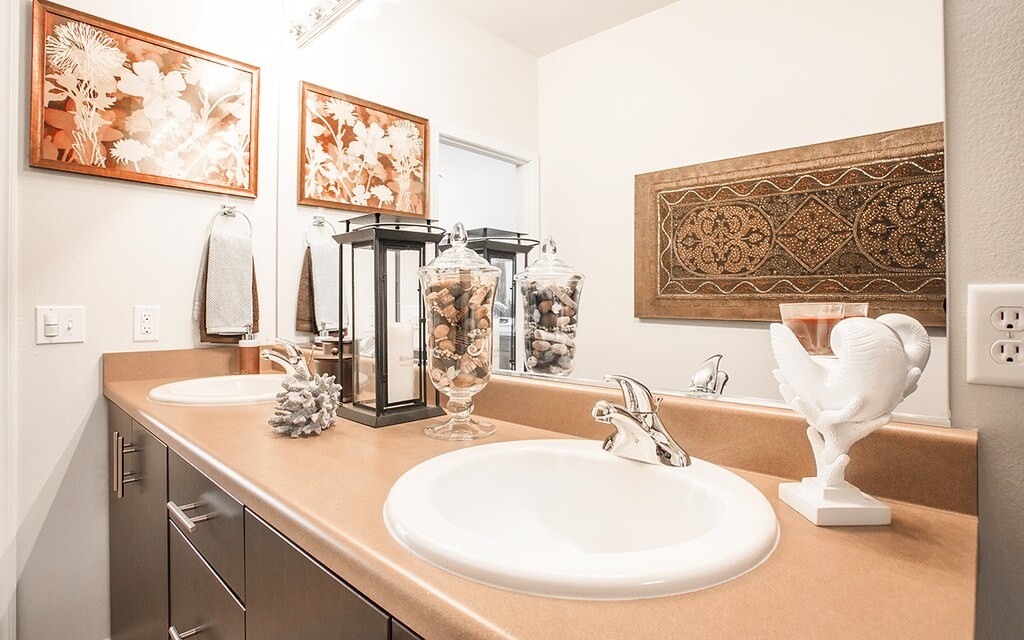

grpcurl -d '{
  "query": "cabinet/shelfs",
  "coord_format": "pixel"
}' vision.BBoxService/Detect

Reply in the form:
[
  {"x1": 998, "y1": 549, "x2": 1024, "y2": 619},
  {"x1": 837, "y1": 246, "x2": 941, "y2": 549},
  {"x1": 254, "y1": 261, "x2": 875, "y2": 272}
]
[{"x1": 106, "y1": 399, "x2": 422, "y2": 640}]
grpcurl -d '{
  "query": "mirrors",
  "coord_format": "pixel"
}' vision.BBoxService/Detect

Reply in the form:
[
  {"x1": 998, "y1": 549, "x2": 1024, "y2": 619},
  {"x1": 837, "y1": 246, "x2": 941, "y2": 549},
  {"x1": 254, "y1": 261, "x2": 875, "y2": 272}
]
[{"x1": 277, "y1": 0, "x2": 951, "y2": 427}]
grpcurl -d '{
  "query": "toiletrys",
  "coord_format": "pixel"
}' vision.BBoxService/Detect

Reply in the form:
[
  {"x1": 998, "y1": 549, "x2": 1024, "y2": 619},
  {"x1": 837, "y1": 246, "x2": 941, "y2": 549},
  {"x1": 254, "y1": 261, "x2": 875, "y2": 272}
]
[
  {"x1": 238, "y1": 323, "x2": 260, "y2": 375},
  {"x1": 314, "y1": 320, "x2": 332, "y2": 348}
]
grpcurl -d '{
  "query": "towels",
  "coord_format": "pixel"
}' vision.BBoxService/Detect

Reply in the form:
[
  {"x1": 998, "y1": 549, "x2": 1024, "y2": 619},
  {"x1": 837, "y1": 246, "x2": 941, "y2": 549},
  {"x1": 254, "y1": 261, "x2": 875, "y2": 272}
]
[
  {"x1": 291, "y1": 242, "x2": 352, "y2": 338},
  {"x1": 191, "y1": 230, "x2": 261, "y2": 344}
]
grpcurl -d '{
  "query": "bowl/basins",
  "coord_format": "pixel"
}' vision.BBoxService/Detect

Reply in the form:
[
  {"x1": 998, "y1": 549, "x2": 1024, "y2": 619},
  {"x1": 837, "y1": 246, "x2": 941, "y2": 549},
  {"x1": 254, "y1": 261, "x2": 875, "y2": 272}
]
[
  {"x1": 779, "y1": 302, "x2": 846, "y2": 355},
  {"x1": 844, "y1": 302, "x2": 869, "y2": 317}
]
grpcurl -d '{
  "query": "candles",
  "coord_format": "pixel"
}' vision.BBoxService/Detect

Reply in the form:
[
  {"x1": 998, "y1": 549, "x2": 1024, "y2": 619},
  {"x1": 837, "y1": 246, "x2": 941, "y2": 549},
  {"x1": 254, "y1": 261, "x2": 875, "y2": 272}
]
[
  {"x1": 386, "y1": 320, "x2": 414, "y2": 402},
  {"x1": 493, "y1": 317, "x2": 500, "y2": 368}
]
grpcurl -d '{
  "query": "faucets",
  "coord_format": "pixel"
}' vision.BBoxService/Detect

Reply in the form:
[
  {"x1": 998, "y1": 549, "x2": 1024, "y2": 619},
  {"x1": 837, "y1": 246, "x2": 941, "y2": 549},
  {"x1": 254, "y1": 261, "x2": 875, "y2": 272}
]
[
  {"x1": 260, "y1": 337, "x2": 311, "y2": 380},
  {"x1": 687, "y1": 354, "x2": 730, "y2": 396},
  {"x1": 592, "y1": 375, "x2": 692, "y2": 468}
]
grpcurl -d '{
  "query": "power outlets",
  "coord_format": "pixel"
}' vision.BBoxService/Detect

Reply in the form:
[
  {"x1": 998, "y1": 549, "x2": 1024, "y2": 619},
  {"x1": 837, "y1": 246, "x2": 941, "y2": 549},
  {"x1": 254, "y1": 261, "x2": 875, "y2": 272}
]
[
  {"x1": 133, "y1": 304, "x2": 159, "y2": 341},
  {"x1": 966, "y1": 284, "x2": 1024, "y2": 386}
]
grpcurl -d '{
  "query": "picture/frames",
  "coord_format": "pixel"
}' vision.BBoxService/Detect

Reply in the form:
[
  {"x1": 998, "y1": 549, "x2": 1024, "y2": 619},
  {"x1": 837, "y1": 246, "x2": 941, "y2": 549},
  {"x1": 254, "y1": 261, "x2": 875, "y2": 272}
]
[
  {"x1": 30, "y1": 0, "x2": 261, "y2": 200},
  {"x1": 297, "y1": 79, "x2": 430, "y2": 219}
]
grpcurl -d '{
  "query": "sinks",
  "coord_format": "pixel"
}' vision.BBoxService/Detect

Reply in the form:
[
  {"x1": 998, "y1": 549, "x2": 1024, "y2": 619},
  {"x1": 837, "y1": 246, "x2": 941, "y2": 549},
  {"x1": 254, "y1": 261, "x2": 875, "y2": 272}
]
[
  {"x1": 147, "y1": 374, "x2": 289, "y2": 407},
  {"x1": 382, "y1": 437, "x2": 780, "y2": 603}
]
